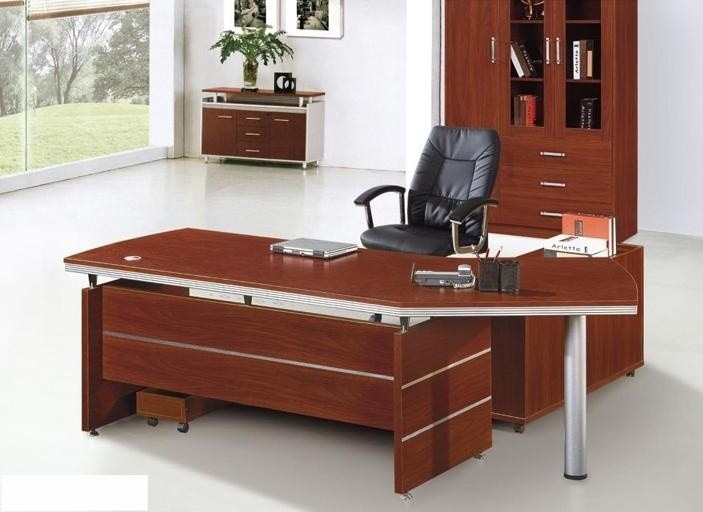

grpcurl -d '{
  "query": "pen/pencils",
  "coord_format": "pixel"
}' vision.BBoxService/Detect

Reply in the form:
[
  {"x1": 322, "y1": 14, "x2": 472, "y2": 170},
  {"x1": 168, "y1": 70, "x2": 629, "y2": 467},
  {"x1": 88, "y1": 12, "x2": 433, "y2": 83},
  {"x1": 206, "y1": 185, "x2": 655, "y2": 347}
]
[
  {"x1": 471, "y1": 244, "x2": 482, "y2": 263},
  {"x1": 492, "y1": 245, "x2": 504, "y2": 264},
  {"x1": 484, "y1": 248, "x2": 490, "y2": 262}
]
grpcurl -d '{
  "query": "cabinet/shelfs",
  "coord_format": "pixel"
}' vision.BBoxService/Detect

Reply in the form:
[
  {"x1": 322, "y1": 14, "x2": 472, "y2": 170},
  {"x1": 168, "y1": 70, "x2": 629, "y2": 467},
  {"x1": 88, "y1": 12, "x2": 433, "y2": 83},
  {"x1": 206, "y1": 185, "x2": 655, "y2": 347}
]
[
  {"x1": 201, "y1": 102, "x2": 236, "y2": 163},
  {"x1": 236, "y1": 104, "x2": 268, "y2": 162},
  {"x1": 268, "y1": 102, "x2": 325, "y2": 169},
  {"x1": 501, "y1": 0, "x2": 637, "y2": 129},
  {"x1": 441, "y1": 0, "x2": 501, "y2": 235},
  {"x1": 503, "y1": 128, "x2": 638, "y2": 245}
]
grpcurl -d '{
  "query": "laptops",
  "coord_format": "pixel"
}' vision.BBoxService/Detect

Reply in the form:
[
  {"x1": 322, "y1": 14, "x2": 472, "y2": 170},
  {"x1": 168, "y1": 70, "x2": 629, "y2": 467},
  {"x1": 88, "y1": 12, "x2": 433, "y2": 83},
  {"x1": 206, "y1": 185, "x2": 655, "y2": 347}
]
[{"x1": 270, "y1": 237, "x2": 358, "y2": 260}]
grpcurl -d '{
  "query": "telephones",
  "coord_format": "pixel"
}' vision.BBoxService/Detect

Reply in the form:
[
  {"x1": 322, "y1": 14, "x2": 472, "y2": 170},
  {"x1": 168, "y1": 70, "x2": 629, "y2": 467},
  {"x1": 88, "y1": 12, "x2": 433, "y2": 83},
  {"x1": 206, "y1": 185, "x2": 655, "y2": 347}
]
[{"x1": 410, "y1": 262, "x2": 475, "y2": 288}]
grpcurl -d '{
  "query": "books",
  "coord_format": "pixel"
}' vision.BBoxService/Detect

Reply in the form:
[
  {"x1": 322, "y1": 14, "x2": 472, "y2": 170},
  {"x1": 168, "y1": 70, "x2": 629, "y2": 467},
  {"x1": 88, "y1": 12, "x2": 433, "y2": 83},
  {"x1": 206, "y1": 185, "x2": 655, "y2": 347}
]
[
  {"x1": 544, "y1": 213, "x2": 616, "y2": 256},
  {"x1": 510, "y1": 40, "x2": 600, "y2": 130}
]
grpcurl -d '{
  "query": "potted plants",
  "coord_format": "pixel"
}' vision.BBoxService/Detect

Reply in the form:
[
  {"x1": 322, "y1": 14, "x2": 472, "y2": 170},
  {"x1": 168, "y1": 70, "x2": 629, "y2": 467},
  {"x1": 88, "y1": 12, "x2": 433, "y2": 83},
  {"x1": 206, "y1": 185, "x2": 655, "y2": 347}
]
[{"x1": 206, "y1": 12, "x2": 294, "y2": 91}]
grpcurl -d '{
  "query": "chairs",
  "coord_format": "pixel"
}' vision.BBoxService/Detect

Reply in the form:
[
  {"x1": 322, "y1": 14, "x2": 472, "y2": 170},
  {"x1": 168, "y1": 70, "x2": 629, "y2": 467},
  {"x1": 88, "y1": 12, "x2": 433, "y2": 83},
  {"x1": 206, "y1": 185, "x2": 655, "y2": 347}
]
[{"x1": 354, "y1": 125, "x2": 501, "y2": 259}]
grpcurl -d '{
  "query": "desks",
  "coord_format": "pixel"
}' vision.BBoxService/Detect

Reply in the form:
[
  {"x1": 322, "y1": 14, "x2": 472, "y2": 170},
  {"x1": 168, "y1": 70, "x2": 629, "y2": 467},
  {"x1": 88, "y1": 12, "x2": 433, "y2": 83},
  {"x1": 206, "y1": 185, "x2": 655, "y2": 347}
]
[
  {"x1": 490, "y1": 244, "x2": 644, "y2": 433},
  {"x1": 60, "y1": 228, "x2": 637, "y2": 493}
]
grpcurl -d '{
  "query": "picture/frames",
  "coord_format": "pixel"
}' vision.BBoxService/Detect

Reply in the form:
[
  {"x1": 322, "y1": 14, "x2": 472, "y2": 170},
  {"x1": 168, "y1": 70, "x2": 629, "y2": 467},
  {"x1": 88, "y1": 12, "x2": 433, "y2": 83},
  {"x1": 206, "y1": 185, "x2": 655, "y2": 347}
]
[
  {"x1": 222, "y1": 0, "x2": 281, "y2": 38},
  {"x1": 284, "y1": 0, "x2": 344, "y2": 39}
]
